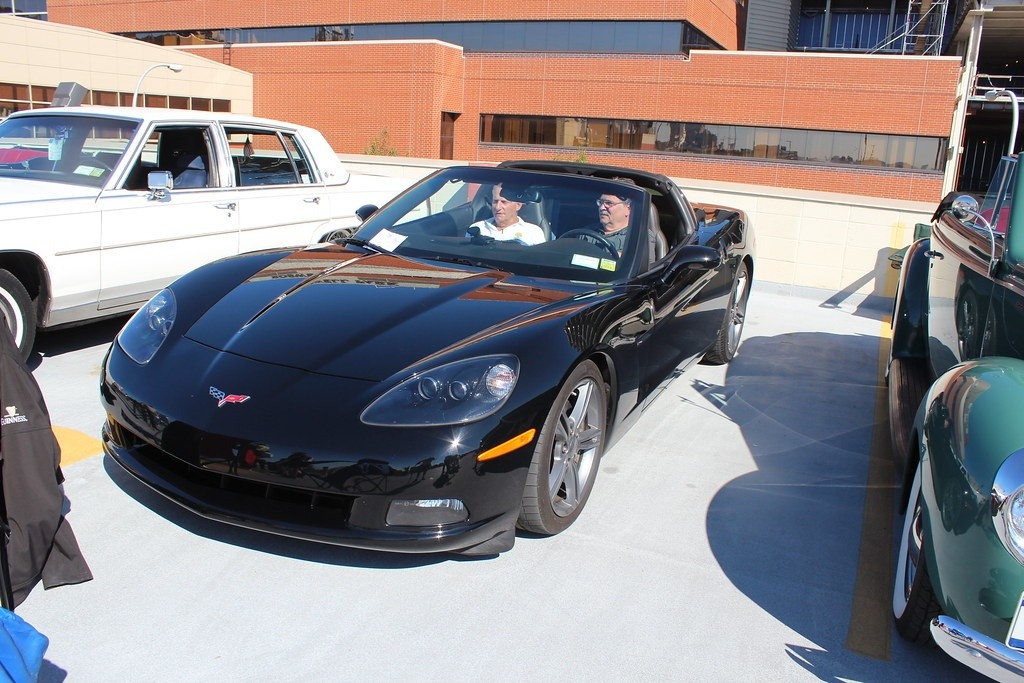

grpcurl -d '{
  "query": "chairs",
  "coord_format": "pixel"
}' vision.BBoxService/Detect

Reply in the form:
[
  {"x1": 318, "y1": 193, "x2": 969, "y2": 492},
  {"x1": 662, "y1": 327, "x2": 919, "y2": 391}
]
[
  {"x1": 168, "y1": 148, "x2": 206, "y2": 189},
  {"x1": 519, "y1": 194, "x2": 550, "y2": 243},
  {"x1": 649, "y1": 204, "x2": 668, "y2": 262}
]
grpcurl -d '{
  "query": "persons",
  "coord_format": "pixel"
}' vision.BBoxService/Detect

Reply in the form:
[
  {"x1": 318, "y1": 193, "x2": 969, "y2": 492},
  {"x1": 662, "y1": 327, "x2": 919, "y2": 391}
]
[
  {"x1": 466, "y1": 183, "x2": 546, "y2": 246},
  {"x1": 577, "y1": 178, "x2": 655, "y2": 264}
]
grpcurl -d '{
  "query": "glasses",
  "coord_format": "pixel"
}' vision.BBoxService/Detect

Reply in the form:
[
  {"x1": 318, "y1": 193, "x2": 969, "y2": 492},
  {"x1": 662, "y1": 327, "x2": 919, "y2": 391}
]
[{"x1": 597, "y1": 199, "x2": 625, "y2": 208}]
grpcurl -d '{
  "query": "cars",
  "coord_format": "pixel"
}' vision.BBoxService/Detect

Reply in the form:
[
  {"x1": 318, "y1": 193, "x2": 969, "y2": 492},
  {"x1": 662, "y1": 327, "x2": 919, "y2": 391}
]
[
  {"x1": 0, "y1": 101, "x2": 366, "y2": 370},
  {"x1": 883, "y1": 148, "x2": 1023, "y2": 683}
]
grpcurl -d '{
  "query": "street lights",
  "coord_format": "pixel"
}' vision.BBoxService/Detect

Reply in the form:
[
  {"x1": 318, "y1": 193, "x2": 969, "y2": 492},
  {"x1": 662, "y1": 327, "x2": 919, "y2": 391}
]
[
  {"x1": 130, "y1": 62, "x2": 183, "y2": 108},
  {"x1": 985, "y1": 87, "x2": 1019, "y2": 160}
]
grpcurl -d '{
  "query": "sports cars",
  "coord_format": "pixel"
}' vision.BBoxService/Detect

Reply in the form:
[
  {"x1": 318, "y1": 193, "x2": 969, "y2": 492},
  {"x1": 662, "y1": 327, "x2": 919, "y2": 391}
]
[{"x1": 97, "y1": 158, "x2": 754, "y2": 557}]
